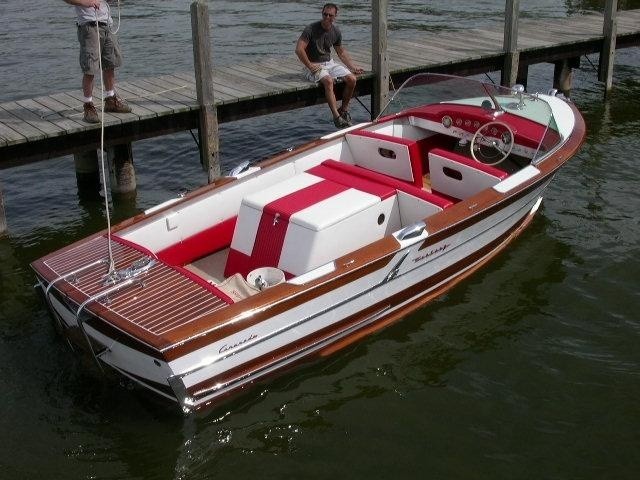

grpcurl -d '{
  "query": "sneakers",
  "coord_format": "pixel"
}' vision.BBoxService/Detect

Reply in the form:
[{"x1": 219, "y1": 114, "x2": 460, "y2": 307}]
[
  {"x1": 82, "y1": 100, "x2": 100, "y2": 124},
  {"x1": 333, "y1": 115, "x2": 348, "y2": 129},
  {"x1": 103, "y1": 96, "x2": 133, "y2": 114},
  {"x1": 338, "y1": 108, "x2": 353, "y2": 126}
]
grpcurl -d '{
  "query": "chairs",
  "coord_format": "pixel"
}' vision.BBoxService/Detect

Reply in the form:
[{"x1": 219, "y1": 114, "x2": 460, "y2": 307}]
[{"x1": 346, "y1": 129, "x2": 508, "y2": 204}]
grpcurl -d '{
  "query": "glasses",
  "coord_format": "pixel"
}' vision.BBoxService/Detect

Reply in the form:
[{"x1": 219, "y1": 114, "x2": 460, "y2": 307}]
[{"x1": 323, "y1": 11, "x2": 335, "y2": 17}]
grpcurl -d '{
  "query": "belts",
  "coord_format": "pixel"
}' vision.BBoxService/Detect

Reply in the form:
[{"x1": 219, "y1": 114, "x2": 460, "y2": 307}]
[{"x1": 90, "y1": 21, "x2": 107, "y2": 27}]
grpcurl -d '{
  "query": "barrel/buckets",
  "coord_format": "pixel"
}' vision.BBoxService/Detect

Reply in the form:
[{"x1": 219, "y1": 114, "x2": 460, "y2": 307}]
[{"x1": 246, "y1": 266, "x2": 286, "y2": 292}]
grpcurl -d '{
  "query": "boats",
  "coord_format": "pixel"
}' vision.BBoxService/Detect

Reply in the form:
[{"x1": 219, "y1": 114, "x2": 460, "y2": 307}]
[{"x1": 27, "y1": 70, "x2": 588, "y2": 421}]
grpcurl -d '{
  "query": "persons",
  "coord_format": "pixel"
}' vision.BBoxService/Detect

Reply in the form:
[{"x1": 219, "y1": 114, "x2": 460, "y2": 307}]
[
  {"x1": 64, "y1": 0, "x2": 131, "y2": 123},
  {"x1": 294, "y1": 3, "x2": 366, "y2": 129}
]
